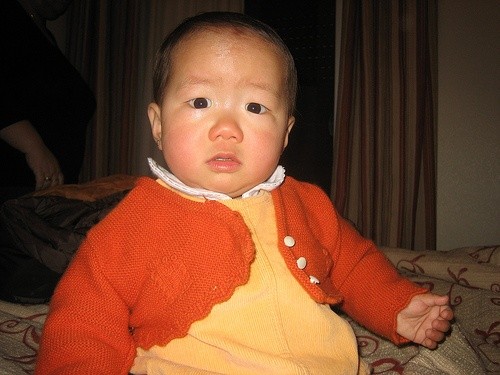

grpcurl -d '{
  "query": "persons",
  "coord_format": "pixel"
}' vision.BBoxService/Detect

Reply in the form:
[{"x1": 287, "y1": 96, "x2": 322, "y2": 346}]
[
  {"x1": 34, "y1": 12, "x2": 455, "y2": 375},
  {"x1": 0, "y1": 0, "x2": 98, "y2": 204}
]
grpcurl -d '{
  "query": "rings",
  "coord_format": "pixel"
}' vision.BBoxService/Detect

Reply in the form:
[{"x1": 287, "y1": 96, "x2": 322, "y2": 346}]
[{"x1": 43, "y1": 175, "x2": 60, "y2": 187}]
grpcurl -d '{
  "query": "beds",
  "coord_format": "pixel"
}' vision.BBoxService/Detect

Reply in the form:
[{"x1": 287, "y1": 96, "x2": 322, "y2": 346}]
[{"x1": 0, "y1": 245, "x2": 500, "y2": 375}]
